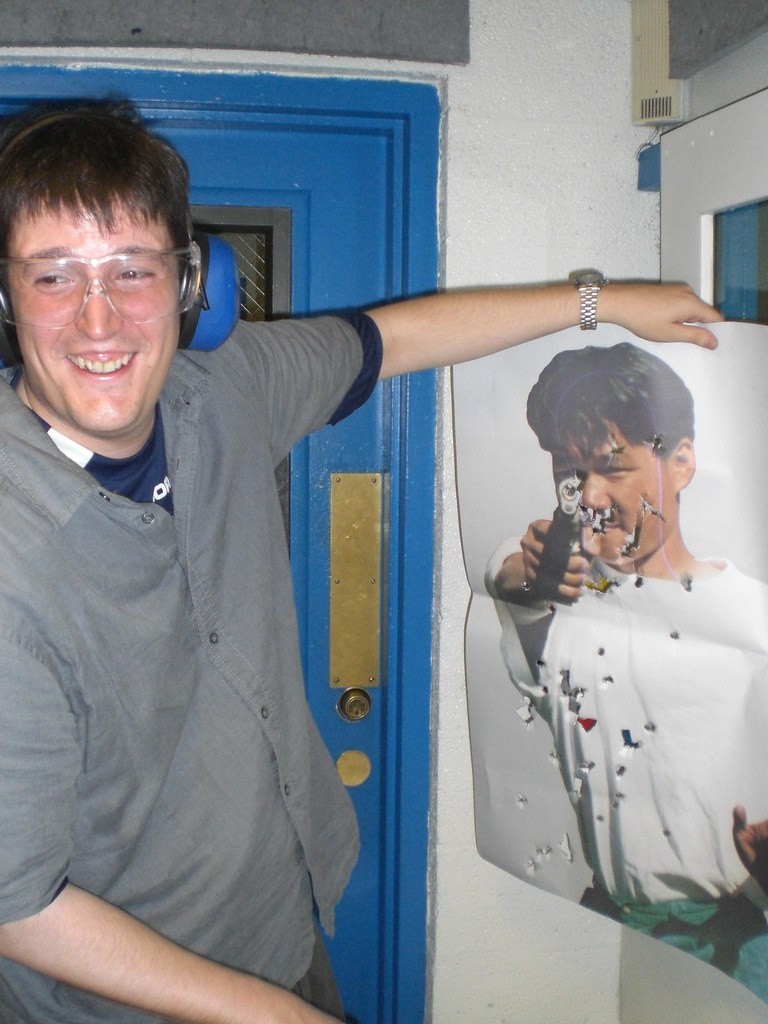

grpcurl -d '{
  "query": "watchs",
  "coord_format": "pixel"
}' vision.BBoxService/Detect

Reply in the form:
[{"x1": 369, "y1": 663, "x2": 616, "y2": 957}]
[{"x1": 572, "y1": 272, "x2": 609, "y2": 331}]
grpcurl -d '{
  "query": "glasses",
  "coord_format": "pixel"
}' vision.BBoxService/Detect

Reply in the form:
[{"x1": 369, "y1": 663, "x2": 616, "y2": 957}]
[{"x1": 0, "y1": 241, "x2": 202, "y2": 329}]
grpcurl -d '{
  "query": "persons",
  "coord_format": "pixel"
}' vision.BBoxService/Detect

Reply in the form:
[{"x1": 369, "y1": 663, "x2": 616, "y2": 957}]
[
  {"x1": 484, "y1": 341, "x2": 768, "y2": 977},
  {"x1": 0, "y1": 90, "x2": 726, "y2": 1024}
]
